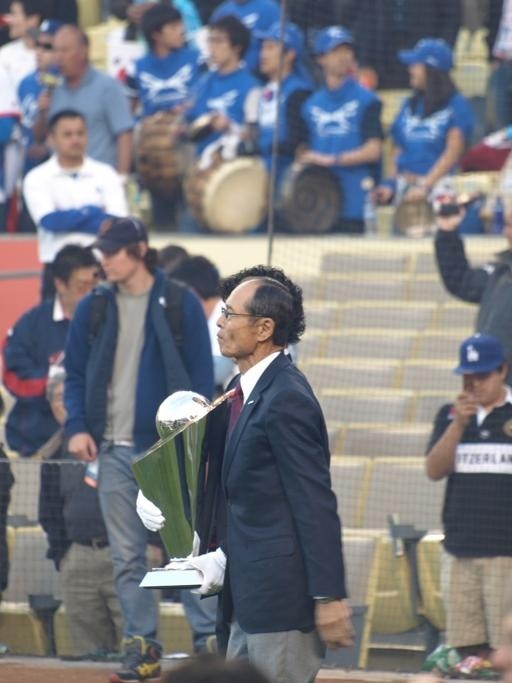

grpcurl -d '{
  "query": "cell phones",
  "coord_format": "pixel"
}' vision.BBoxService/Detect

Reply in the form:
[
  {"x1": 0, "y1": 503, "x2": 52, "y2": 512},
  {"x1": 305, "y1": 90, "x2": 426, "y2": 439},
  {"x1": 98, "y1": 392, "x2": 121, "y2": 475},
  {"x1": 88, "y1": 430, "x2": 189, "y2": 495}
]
[{"x1": 464, "y1": 383, "x2": 473, "y2": 392}]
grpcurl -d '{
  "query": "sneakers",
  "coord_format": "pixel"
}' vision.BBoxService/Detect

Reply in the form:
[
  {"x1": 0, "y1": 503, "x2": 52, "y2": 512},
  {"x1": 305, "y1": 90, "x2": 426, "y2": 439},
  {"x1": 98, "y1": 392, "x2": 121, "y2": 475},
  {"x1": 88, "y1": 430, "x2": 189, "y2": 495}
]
[{"x1": 112, "y1": 635, "x2": 165, "y2": 677}]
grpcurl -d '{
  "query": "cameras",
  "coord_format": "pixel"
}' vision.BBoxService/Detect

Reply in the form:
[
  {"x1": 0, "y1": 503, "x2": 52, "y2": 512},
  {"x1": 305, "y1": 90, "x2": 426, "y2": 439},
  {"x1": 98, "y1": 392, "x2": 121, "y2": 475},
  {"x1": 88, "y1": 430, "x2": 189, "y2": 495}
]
[{"x1": 434, "y1": 203, "x2": 458, "y2": 216}]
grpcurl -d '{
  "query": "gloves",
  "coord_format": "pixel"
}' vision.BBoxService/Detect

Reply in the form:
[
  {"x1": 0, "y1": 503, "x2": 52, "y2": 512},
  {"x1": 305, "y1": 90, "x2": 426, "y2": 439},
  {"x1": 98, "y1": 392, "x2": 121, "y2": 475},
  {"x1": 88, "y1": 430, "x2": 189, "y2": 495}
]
[
  {"x1": 133, "y1": 487, "x2": 167, "y2": 534},
  {"x1": 165, "y1": 545, "x2": 230, "y2": 601}
]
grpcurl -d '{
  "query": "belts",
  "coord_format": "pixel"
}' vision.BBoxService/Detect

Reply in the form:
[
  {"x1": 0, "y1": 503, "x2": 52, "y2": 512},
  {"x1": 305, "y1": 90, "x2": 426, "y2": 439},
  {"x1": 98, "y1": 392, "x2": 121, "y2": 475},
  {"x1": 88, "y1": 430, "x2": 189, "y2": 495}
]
[{"x1": 67, "y1": 537, "x2": 112, "y2": 552}]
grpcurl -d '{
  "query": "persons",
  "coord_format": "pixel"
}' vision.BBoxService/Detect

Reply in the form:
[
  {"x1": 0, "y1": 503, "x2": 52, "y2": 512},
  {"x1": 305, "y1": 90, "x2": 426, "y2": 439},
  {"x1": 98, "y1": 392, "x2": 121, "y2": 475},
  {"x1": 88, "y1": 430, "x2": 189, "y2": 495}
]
[
  {"x1": 1, "y1": 215, "x2": 356, "y2": 681},
  {"x1": 21, "y1": 109, "x2": 127, "y2": 303},
  {"x1": 426, "y1": 200, "x2": 511, "y2": 681},
  {"x1": 0, "y1": 0, "x2": 510, "y2": 233}
]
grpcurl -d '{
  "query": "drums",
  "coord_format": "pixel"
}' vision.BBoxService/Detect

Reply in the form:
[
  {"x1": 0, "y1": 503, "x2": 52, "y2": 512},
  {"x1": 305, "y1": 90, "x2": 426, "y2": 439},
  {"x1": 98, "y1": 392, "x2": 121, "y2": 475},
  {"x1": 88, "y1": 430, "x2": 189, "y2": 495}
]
[
  {"x1": 274, "y1": 159, "x2": 341, "y2": 235},
  {"x1": 183, "y1": 149, "x2": 270, "y2": 233},
  {"x1": 135, "y1": 111, "x2": 195, "y2": 191}
]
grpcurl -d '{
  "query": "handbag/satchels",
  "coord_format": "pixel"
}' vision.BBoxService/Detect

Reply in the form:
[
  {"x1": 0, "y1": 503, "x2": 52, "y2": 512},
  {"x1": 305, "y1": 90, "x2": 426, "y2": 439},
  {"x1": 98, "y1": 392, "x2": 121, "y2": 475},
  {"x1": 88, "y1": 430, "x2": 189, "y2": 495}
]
[{"x1": 4, "y1": 399, "x2": 64, "y2": 462}]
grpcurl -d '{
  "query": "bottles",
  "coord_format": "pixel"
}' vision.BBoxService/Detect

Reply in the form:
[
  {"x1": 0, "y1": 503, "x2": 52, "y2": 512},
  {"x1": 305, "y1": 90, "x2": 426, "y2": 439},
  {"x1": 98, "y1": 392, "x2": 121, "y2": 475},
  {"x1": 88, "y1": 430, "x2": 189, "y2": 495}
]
[{"x1": 357, "y1": 176, "x2": 455, "y2": 238}]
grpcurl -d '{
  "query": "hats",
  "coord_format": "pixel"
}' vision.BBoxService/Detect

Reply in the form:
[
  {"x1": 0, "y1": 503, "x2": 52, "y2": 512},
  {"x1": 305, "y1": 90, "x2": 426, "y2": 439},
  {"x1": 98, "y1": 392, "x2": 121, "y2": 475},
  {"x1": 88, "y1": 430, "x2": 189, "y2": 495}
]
[
  {"x1": 397, "y1": 36, "x2": 457, "y2": 72},
  {"x1": 252, "y1": 20, "x2": 306, "y2": 57},
  {"x1": 26, "y1": 17, "x2": 63, "y2": 38},
  {"x1": 309, "y1": 26, "x2": 358, "y2": 56},
  {"x1": 87, "y1": 213, "x2": 149, "y2": 252},
  {"x1": 452, "y1": 333, "x2": 505, "y2": 377}
]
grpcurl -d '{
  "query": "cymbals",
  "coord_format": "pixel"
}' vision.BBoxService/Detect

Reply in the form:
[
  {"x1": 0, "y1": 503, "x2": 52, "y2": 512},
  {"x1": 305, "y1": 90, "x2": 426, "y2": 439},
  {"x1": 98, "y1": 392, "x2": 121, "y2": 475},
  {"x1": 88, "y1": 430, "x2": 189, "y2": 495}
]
[{"x1": 395, "y1": 199, "x2": 436, "y2": 236}]
